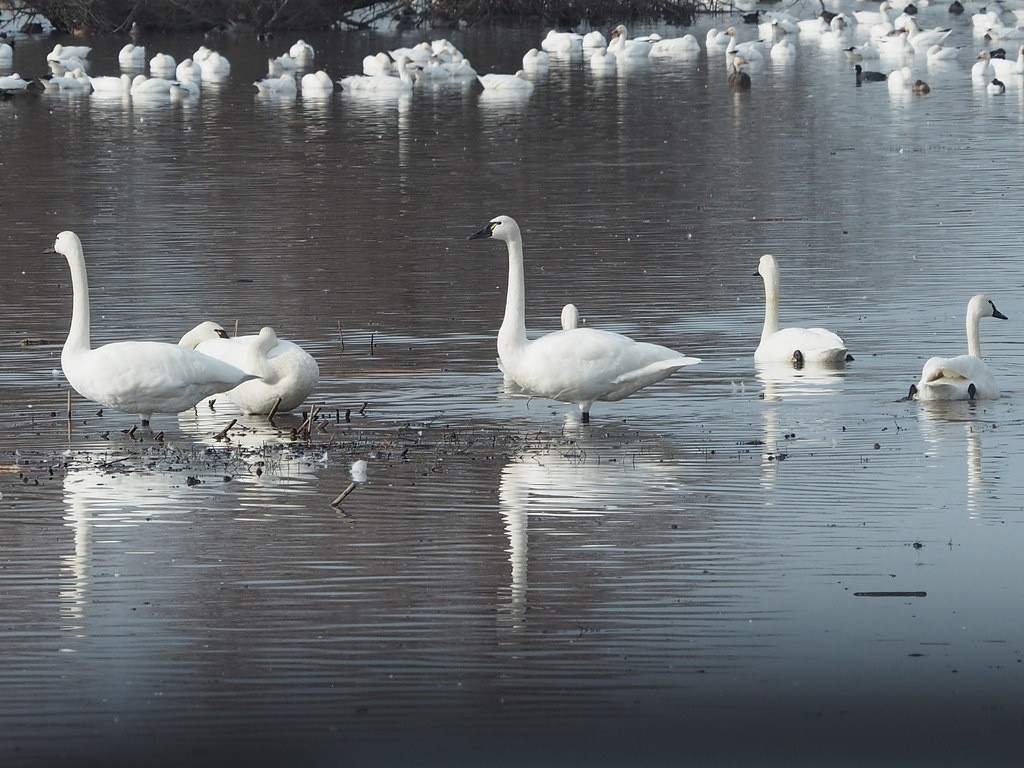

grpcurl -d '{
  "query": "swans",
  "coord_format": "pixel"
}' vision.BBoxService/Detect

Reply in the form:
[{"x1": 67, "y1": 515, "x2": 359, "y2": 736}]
[
  {"x1": 901, "y1": 294, "x2": 1009, "y2": 402},
  {"x1": 752, "y1": 253, "x2": 854, "y2": 364},
  {"x1": 43, "y1": 231, "x2": 264, "y2": 427},
  {"x1": 469, "y1": 215, "x2": 704, "y2": 422},
  {"x1": 0, "y1": 1, "x2": 1024, "y2": 98},
  {"x1": 178, "y1": 321, "x2": 320, "y2": 415}
]
